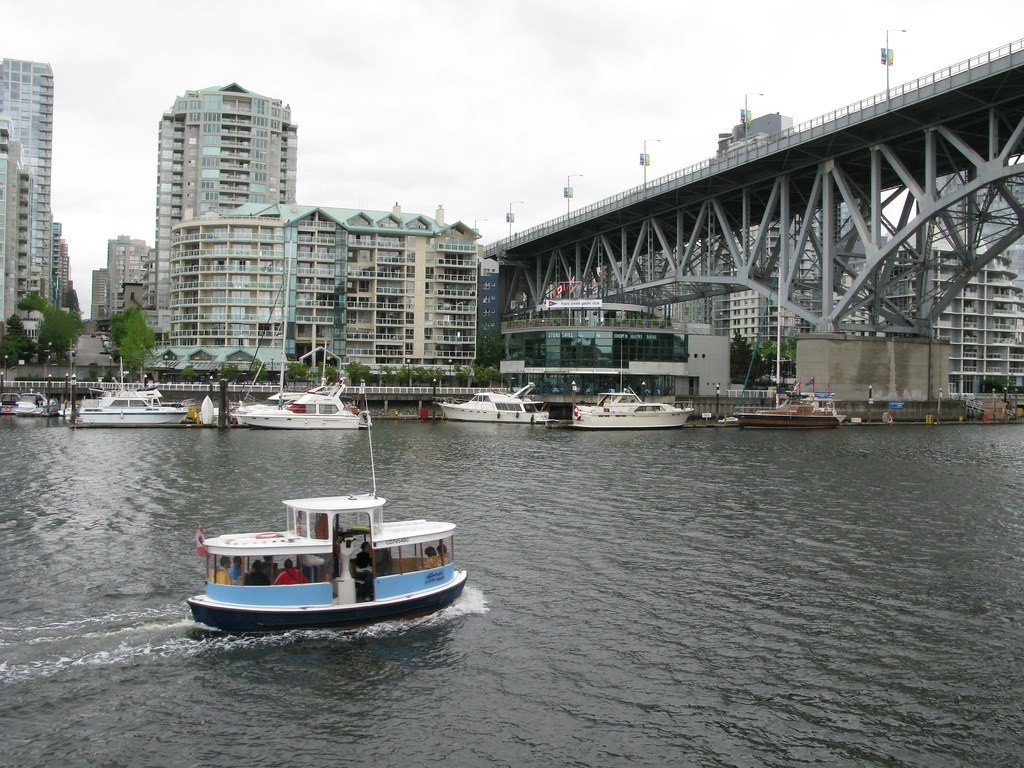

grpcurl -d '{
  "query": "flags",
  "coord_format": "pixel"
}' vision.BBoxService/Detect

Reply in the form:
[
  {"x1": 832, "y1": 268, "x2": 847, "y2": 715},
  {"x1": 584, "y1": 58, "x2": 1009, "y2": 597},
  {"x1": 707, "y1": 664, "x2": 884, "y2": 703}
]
[
  {"x1": 573, "y1": 403, "x2": 583, "y2": 421},
  {"x1": 196, "y1": 523, "x2": 212, "y2": 558},
  {"x1": 794, "y1": 377, "x2": 815, "y2": 391},
  {"x1": 555, "y1": 284, "x2": 566, "y2": 296}
]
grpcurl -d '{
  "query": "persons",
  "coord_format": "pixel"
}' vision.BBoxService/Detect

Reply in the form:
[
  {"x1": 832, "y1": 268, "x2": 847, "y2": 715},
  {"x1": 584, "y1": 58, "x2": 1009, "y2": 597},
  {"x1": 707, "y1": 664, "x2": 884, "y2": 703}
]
[
  {"x1": 278, "y1": 396, "x2": 283, "y2": 410},
  {"x1": 1005, "y1": 401, "x2": 1013, "y2": 420},
  {"x1": 315, "y1": 515, "x2": 339, "y2": 578},
  {"x1": 419, "y1": 543, "x2": 450, "y2": 572},
  {"x1": 211, "y1": 555, "x2": 309, "y2": 586},
  {"x1": 354, "y1": 542, "x2": 391, "y2": 601}
]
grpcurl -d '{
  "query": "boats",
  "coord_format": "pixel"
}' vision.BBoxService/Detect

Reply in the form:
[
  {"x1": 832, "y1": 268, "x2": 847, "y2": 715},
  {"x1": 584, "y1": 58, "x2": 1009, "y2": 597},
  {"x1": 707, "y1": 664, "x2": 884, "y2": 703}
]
[
  {"x1": 436, "y1": 385, "x2": 550, "y2": 424},
  {"x1": 232, "y1": 392, "x2": 300, "y2": 412},
  {"x1": 0, "y1": 392, "x2": 196, "y2": 422},
  {"x1": 186, "y1": 493, "x2": 470, "y2": 632},
  {"x1": 573, "y1": 389, "x2": 695, "y2": 427},
  {"x1": 733, "y1": 389, "x2": 844, "y2": 426}
]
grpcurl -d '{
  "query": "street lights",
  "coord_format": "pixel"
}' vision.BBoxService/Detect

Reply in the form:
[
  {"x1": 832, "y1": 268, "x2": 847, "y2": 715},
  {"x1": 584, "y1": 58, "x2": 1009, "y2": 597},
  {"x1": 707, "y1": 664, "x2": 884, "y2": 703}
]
[
  {"x1": 744, "y1": 92, "x2": 763, "y2": 138},
  {"x1": 474, "y1": 218, "x2": 488, "y2": 232},
  {"x1": 5, "y1": 355, "x2": 8, "y2": 381},
  {"x1": 164, "y1": 355, "x2": 168, "y2": 382},
  {"x1": 567, "y1": 174, "x2": 583, "y2": 220},
  {"x1": 885, "y1": 28, "x2": 907, "y2": 109},
  {"x1": 270, "y1": 357, "x2": 274, "y2": 381},
  {"x1": 509, "y1": 201, "x2": 526, "y2": 240},
  {"x1": 407, "y1": 358, "x2": 410, "y2": 385},
  {"x1": 644, "y1": 139, "x2": 660, "y2": 193},
  {"x1": 449, "y1": 359, "x2": 453, "y2": 384}
]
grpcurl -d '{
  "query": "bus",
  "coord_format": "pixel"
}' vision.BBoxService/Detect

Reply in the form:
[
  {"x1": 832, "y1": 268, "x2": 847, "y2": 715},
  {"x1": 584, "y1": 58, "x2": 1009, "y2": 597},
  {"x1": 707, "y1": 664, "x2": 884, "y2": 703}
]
[{"x1": 757, "y1": 360, "x2": 795, "y2": 385}]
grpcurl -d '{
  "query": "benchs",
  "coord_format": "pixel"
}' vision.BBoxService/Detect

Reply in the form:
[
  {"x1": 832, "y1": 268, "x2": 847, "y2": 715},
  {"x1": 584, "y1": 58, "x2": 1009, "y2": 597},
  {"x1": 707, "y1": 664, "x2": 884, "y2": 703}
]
[{"x1": 349, "y1": 557, "x2": 429, "y2": 597}]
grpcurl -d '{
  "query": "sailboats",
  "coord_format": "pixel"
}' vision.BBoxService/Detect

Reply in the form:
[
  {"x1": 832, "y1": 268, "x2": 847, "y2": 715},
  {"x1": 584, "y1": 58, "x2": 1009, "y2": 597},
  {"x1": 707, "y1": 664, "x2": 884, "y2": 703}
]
[{"x1": 228, "y1": 240, "x2": 367, "y2": 430}]
